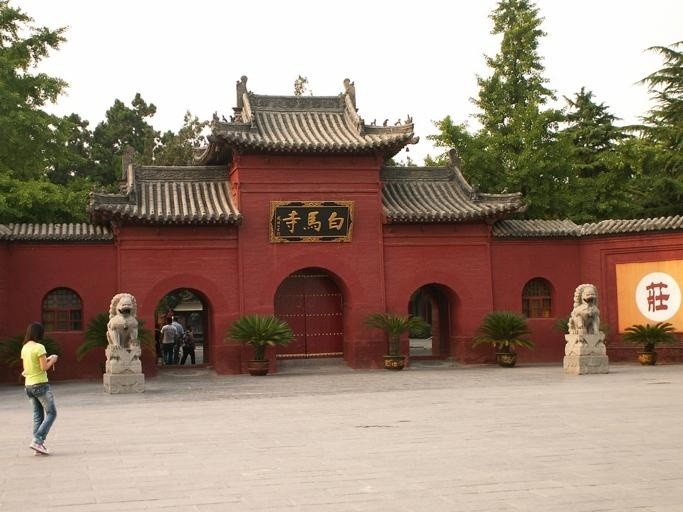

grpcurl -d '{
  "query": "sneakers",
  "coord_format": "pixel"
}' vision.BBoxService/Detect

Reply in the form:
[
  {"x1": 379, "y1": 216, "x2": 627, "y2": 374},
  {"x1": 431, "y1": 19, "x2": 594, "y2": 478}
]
[{"x1": 29, "y1": 442, "x2": 48, "y2": 455}]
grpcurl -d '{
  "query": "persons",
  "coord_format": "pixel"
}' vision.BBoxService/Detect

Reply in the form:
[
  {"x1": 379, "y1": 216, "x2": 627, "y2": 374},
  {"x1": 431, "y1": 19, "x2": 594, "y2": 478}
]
[
  {"x1": 21, "y1": 322, "x2": 58, "y2": 455},
  {"x1": 154, "y1": 316, "x2": 195, "y2": 365}
]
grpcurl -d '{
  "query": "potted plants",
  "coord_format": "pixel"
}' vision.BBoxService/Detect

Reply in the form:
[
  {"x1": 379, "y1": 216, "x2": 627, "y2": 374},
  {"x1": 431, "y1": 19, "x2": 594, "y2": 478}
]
[
  {"x1": 621, "y1": 322, "x2": 678, "y2": 366},
  {"x1": 363, "y1": 312, "x2": 433, "y2": 370},
  {"x1": 469, "y1": 310, "x2": 535, "y2": 366},
  {"x1": 223, "y1": 314, "x2": 293, "y2": 375}
]
